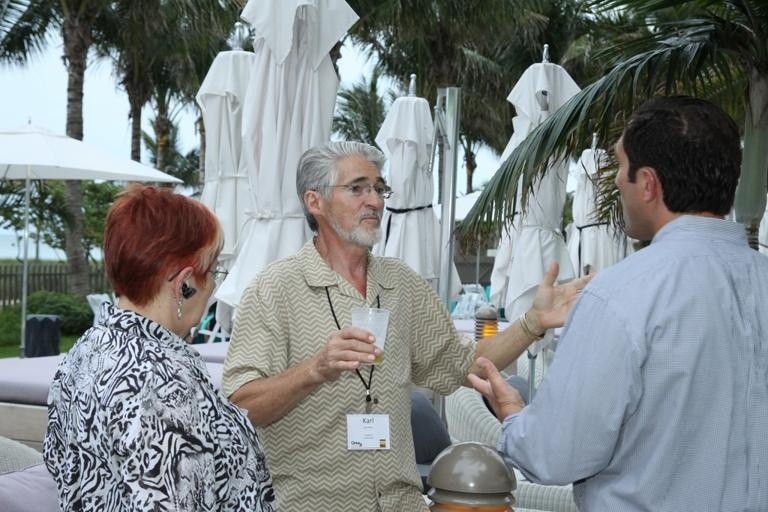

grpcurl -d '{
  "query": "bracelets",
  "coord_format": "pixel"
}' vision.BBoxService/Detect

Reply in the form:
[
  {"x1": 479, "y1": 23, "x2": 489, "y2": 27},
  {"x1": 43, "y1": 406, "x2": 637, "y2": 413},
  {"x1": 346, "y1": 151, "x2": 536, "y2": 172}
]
[{"x1": 520, "y1": 312, "x2": 544, "y2": 341}]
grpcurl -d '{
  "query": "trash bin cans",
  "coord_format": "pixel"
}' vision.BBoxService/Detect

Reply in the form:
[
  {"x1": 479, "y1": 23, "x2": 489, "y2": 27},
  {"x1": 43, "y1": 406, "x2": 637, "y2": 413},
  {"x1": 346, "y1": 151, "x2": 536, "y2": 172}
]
[
  {"x1": 500, "y1": 308, "x2": 505, "y2": 318},
  {"x1": 26, "y1": 315, "x2": 61, "y2": 356}
]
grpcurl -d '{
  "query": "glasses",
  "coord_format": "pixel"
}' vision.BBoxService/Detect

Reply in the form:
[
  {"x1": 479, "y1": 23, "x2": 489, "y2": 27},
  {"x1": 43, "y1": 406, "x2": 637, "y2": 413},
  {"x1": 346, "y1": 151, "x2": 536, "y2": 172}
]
[
  {"x1": 169, "y1": 264, "x2": 229, "y2": 282},
  {"x1": 319, "y1": 183, "x2": 393, "y2": 199}
]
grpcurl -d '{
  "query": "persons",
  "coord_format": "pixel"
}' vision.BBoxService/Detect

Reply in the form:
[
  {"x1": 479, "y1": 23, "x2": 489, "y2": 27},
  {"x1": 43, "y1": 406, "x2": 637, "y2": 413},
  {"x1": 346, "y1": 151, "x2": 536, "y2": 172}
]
[
  {"x1": 468, "y1": 93, "x2": 768, "y2": 512},
  {"x1": 220, "y1": 142, "x2": 597, "y2": 512},
  {"x1": 44, "y1": 186, "x2": 278, "y2": 512}
]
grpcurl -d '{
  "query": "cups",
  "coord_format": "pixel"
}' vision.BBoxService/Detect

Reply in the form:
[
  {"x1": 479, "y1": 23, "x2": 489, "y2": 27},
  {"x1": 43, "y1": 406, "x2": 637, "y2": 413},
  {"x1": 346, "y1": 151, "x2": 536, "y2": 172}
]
[{"x1": 352, "y1": 306, "x2": 389, "y2": 367}]
